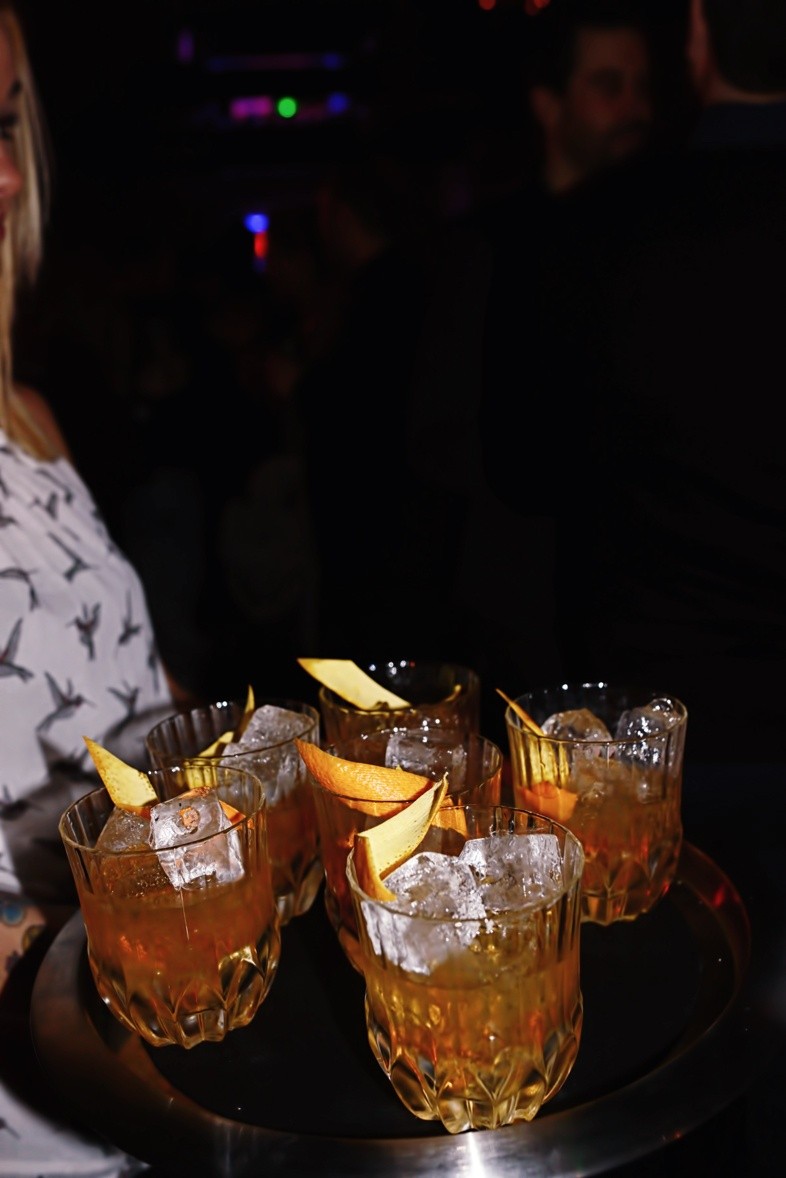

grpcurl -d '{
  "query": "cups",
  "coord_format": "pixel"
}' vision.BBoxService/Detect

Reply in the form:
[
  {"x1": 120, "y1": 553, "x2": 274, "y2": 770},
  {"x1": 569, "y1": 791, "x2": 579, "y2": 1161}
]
[
  {"x1": 57, "y1": 766, "x2": 283, "y2": 1051},
  {"x1": 318, "y1": 661, "x2": 478, "y2": 766},
  {"x1": 308, "y1": 729, "x2": 507, "y2": 975},
  {"x1": 145, "y1": 698, "x2": 321, "y2": 927},
  {"x1": 504, "y1": 682, "x2": 690, "y2": 926},
  {"x1": 344, "y1": 804, "x2": 584, "y2": 1133}
]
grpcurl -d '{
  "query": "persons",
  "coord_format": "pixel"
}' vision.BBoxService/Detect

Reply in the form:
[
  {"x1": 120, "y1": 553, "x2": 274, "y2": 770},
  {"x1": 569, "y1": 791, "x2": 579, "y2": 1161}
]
[
  {"x1": 1, "y1": 6, "x2": 179, "y2": 1178},
  {"x1": 225, "y1": 0, "x2": 786, "y2": 661}
]
